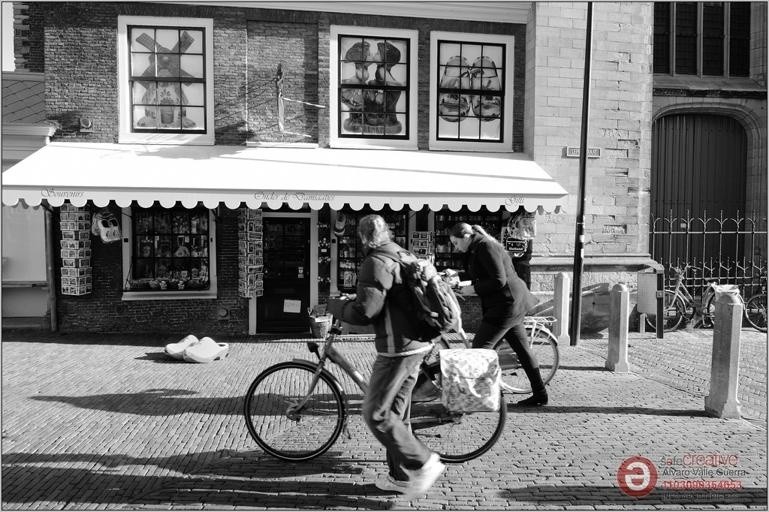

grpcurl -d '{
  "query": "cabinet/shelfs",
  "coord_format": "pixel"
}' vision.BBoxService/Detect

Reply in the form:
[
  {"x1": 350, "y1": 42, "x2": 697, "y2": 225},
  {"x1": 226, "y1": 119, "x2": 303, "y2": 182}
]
[
  {"x1": 434, "y1": 210, "x2": 500, "y2": 275},
  {"x1": 338, "y1": 209, "x2": 407, "y2": 289},
  {"x1": 129, "y1": 208, "x2": 209, "y2": 290}
]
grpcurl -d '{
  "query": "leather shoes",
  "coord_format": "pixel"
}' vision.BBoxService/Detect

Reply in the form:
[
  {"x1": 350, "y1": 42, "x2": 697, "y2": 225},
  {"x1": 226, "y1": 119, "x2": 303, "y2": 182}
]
[{"x1": 165, "y1": 333, "x2": 229, "y2": 363}]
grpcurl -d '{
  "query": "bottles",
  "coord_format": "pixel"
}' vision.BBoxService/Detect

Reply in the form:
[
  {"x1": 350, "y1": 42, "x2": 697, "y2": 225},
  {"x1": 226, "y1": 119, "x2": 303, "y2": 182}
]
[{"x1": 191, "y1": 217, "x2": 198, "y2": 234}]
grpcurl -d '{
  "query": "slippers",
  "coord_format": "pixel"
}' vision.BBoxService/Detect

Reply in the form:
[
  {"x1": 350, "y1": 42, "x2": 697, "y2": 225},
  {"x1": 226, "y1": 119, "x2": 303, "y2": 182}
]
[{"x1": 438, "y1": 54, "x2": 502, "y2": 121}]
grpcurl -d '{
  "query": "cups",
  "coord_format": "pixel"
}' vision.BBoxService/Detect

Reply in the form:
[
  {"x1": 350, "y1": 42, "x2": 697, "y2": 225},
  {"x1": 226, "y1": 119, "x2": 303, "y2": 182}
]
[{"x1": 344, "y1": 247, "x2": 355, "y2": 258}]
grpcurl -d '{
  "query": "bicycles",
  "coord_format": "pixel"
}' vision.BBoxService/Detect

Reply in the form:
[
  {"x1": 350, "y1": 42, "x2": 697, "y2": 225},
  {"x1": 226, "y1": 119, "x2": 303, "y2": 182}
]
[
  {"x1": 243, "y1": 291, "x2": 507, "y2": 464},
  {"x1": 644, "y1": 264, "x2": 746, "y2": 333},
  {"x1": 744, "y1": 271, "x2": 767, "y2": 334},
  {"x1": 409, "y1": 271, "x2": 561, "y2": 403}
]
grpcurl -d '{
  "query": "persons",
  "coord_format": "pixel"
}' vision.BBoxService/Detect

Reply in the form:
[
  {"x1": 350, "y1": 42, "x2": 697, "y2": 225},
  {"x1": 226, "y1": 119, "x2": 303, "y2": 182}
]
[
  {"x1": 449, "y1": 222, "x2": 548, "y2": 406},
  {"x1": 325, "y1": 215, "x2": 447, "y2": 504}
]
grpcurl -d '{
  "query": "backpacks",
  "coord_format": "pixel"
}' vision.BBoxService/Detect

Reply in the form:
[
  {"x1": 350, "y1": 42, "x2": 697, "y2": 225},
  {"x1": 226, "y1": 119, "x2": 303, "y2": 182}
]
[
  {"x1": 438, "y1": 348, "x2": 503, "y2": 412},
  {"x1": 374, "y1": 247, "x2": 461, "y2": 343}
]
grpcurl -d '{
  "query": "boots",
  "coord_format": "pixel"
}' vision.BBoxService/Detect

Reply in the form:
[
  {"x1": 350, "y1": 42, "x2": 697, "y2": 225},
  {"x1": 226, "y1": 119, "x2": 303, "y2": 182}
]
[{"x1": 517, "y1": 367, "x2": 549, "y2": 407}]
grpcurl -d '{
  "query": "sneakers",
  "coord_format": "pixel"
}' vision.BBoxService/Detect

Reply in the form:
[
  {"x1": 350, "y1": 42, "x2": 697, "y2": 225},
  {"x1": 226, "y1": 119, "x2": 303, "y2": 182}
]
[{"x1": 375, "y1": 453, "x2": 446, "y2": 499}]
